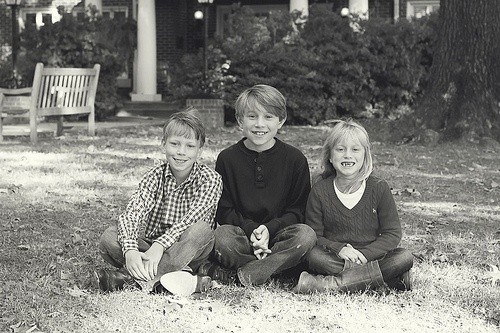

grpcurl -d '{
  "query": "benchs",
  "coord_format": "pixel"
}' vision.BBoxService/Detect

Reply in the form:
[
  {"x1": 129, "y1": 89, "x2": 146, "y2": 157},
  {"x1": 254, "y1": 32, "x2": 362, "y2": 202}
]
[{"x1": 0, "y1": 63, "x2": 101, "y2": 143}]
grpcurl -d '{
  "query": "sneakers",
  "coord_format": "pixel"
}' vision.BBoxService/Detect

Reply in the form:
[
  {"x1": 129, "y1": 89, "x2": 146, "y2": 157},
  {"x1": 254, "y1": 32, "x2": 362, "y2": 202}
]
[
  {"x1": 290, "y1": 263, "x2": 310, "y2": 280},
  {"x1": 206, "y1": 263, "x2": 237, "y2": 285}
]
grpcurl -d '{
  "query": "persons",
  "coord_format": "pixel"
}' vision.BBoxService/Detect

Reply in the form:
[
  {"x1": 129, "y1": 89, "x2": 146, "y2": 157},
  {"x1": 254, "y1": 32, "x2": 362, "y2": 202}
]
[
  {"x1": 199, "y1": 85, "x2": 317, "y2": 288},
  {"x1": 94, "y1": 106, "x2": 223, "y2": 296},
  {"x1": 298, "y1": 117, "x2": 414, "y2": 295}
]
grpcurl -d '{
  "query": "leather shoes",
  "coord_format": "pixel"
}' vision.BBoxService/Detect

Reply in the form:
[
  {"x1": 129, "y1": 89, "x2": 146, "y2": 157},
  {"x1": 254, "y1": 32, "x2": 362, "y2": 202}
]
[
  {"x1": 97, "y1": 268, "x2": 125, "y2": 292},
  {"x1": 159, "y1": 271, "x2": 212, "y2": 296}
]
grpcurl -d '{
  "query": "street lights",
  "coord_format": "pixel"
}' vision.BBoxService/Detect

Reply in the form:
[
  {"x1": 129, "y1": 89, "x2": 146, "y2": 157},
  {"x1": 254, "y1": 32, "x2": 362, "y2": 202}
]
[
  {"x1": 4, "y1": 0, "x2": 26, "y2": 89},
  {"x1": 196, "y1": 0, "x2": 216, "y2": 84}
]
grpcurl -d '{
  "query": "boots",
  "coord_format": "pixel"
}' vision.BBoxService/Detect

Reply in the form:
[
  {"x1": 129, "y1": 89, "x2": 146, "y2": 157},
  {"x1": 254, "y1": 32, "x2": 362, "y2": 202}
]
[
  {"x1": 294, "y1": 260, "x2": 388, "y2": 294},
  {"x1": 335, "y1": 259, "x2": 410, "y2": 291}
]
